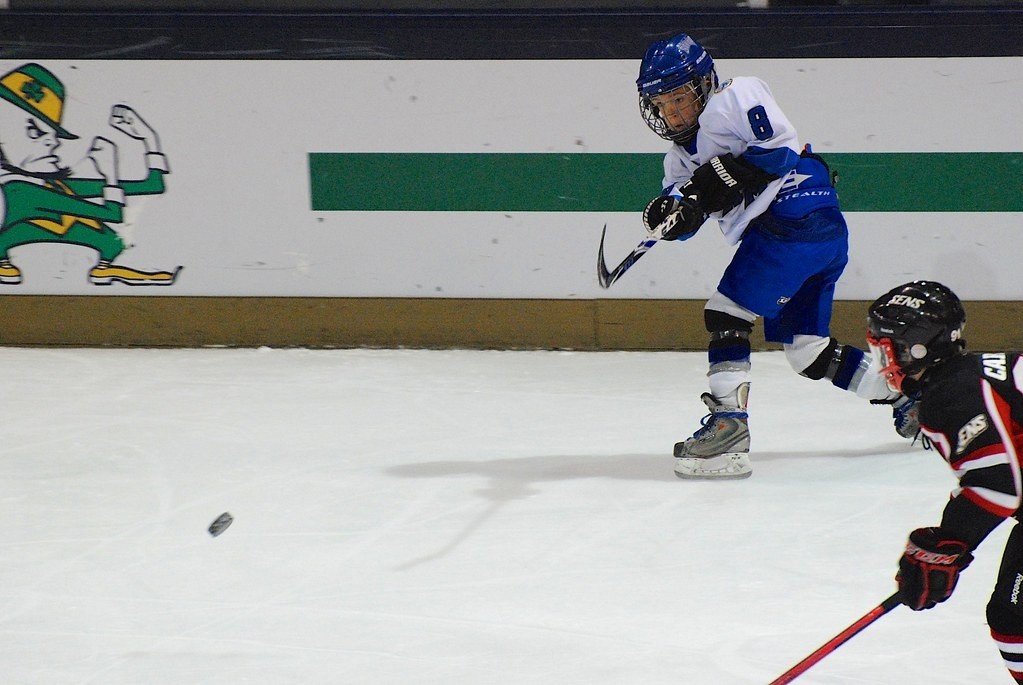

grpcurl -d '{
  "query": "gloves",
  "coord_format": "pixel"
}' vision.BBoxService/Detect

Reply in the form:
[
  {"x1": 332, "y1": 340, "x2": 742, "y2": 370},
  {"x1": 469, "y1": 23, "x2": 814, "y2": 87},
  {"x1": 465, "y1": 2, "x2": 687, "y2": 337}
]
[
  {"x1": 683, "y1": 154, "x2": 758, "y2": 211},
  {"x1": 644, "y1": 196, "x2": 696, "y2": 243},
  {"x1": 897, "y1": 526, "x2": 973, "y2": 610}
]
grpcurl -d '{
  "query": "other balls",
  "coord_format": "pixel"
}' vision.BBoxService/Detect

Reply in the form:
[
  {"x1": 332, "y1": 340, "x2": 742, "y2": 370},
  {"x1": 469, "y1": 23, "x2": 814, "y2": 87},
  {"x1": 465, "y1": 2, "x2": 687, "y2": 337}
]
[{"x1": 209, "y1": 511, "x2": 234, "y2": 537}]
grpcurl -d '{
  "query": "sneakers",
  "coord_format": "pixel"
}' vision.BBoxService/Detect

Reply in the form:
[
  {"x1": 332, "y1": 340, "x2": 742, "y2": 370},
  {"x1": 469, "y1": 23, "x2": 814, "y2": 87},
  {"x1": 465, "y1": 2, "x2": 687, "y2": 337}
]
[
  {"x1": 671, "y1": 383, "x2": 751, "y2": 479},
  {"x1": 868, "y1": 391, "x2": 934, "y2": 441}
]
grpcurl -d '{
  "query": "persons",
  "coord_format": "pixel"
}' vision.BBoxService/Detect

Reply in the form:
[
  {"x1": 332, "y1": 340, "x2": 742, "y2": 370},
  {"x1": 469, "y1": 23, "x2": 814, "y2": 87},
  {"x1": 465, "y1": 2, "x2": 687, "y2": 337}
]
[
  {"x1": 877, "y1": 294, "x2": 1022, "y2": 685},
  {"x1": 639, "y1": 51, "x2": 927, "y2": 479},
  {"x1": 0, "y1": 61, "x2": 176, "y2": 285}
]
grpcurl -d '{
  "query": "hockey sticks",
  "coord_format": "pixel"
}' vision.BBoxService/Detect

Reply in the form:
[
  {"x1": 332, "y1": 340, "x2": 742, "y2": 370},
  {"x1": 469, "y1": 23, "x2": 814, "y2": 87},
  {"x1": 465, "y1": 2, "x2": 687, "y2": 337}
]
[
  {"x1": 768, "y1": 591, "x2": 905, "y2": 685},
  {"x1": 598, "y1": 191, "x2": 698, "y2": 289}
]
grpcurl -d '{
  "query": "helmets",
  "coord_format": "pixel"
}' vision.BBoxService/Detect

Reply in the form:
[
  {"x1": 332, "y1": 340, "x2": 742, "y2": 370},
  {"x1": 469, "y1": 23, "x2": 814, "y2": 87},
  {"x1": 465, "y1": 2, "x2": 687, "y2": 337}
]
[
  {"x1": 865, "y1": 280, "x2": 967, "y2": 391},
  {"x1": 637, "y1": 35, "x2": 716, "y2": 145}
]
